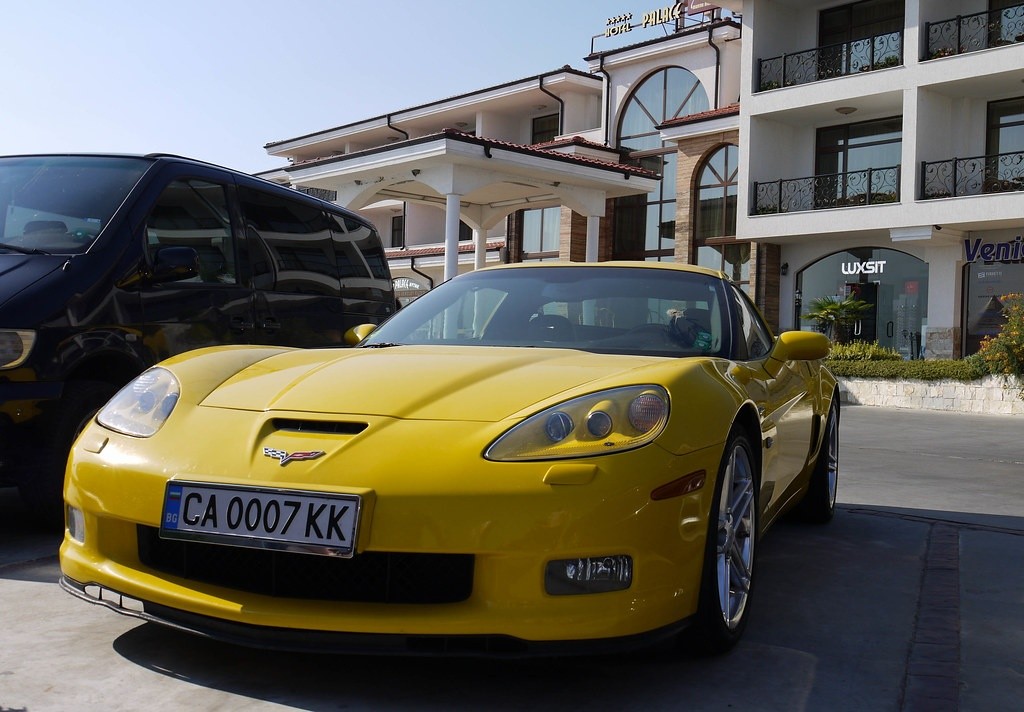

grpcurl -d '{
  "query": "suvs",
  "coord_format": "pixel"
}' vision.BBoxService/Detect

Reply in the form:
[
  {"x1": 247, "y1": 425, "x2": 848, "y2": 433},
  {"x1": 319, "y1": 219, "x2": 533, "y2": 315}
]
[{"x1": 0, "y1": 152, "x2": 406, "y2": 536}]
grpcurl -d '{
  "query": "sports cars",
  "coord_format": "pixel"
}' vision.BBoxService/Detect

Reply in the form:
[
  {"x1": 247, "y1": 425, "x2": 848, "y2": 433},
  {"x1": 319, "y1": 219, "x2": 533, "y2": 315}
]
[{"x1": 58, "y1": 260, "x2": 842, "y2": 657}]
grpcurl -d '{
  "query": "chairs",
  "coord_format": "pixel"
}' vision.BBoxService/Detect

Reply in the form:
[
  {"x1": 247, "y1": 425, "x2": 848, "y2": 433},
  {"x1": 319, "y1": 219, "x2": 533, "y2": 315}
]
[
  {"x1": 524, "y1": 315, "x2": 575, "y2": 342},
  {"x1": 668, "y1": 308, "x2": 712, "y2": 350}
]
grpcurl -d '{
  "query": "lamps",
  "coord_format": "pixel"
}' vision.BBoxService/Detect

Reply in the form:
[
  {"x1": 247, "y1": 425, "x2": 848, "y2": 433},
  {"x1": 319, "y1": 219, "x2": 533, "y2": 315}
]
[
  {"x1": 781, "y1": 262, "x2": 788, "y2": 274},
  {"x1": 836, "y1": 107, "x2": 857, "y2": 114}
]
